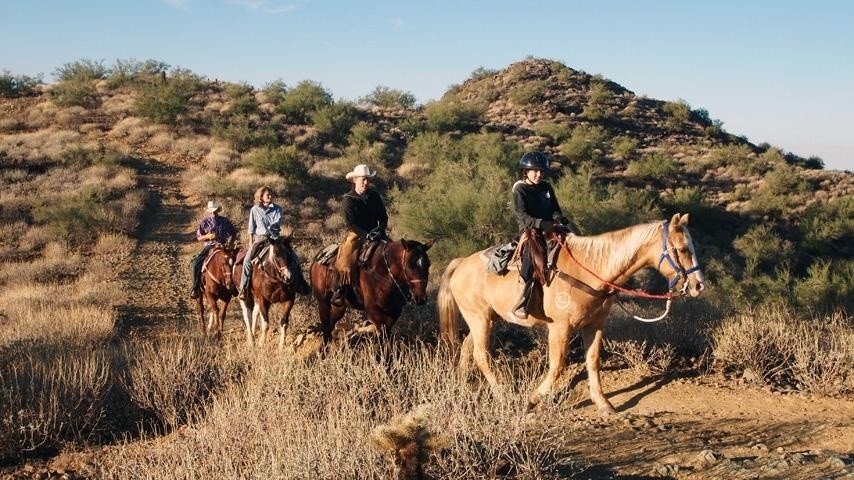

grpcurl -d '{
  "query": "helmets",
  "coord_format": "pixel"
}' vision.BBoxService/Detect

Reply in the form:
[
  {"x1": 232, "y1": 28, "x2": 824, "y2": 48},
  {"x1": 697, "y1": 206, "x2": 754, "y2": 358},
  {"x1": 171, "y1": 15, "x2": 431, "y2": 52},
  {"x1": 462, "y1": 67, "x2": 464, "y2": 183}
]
[{"x1": 518, "y1": 150, "x2": 552, "y2": 172}]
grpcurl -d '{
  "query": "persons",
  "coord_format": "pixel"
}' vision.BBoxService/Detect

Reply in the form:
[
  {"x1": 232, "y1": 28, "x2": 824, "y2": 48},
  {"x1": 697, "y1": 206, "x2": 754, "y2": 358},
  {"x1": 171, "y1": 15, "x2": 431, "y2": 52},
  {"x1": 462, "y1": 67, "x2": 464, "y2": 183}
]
[
  {"x1": 510, "y1": 151, "x2": 582, "y2": 320},
  {"x1": 188, "y1": 200, "x2": 236, "y2": 299},
  {"x1": 238, "y1": 185, "x2": 312, "y2": 303},
  {"x1": 328, "y1": 165, "x2": 388, "y2": 309}
]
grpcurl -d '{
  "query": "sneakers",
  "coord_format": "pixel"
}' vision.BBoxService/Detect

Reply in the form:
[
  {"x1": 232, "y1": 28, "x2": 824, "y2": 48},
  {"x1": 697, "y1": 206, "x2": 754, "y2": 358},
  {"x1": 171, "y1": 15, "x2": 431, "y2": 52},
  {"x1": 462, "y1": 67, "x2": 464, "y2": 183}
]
[
  {"x1": 190, "y1": 288, "x2": 200, "y2": 298},
  {"x1": 331, "y1": 288, "x2": 345, "y2": 306},
  {"x1": 238, "y1": 289, "x2": 248, "y2": 300}
]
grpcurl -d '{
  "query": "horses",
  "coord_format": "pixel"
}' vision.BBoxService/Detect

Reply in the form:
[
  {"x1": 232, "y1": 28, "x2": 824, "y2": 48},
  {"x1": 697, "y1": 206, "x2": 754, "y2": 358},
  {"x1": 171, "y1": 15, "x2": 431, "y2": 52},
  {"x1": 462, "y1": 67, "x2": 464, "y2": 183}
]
[
  {"x1": 309, "y1": 236, "x2": 437, "y2": 370},
  {"x1": 231, "y1": 228, "x2": 298, "y2": 350},
  {"x1": 196, "y1": 237, "x2": 241, "y2": 342},
  {"x1": 435, "y1": 211, "x2": 706, "y2": 417}
]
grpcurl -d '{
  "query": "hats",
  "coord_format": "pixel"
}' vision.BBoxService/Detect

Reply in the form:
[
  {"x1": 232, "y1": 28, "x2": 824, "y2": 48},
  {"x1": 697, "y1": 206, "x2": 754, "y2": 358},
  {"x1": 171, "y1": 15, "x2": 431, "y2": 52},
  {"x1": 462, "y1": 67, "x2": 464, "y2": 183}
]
[
  {"x1": 203, "y1": 200, "x2": 222, "y2": 214},
  {"x1": 345, "y1": 164, "x2": 377, "y2": 180}
]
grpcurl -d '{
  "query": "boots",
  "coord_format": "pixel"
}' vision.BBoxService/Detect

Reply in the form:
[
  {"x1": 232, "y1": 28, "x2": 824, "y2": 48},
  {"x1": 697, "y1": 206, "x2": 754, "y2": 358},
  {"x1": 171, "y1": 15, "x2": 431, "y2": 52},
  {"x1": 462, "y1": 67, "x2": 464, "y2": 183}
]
[{"x1": 512, "y1": 273, "x2": 534, "y2": 320}]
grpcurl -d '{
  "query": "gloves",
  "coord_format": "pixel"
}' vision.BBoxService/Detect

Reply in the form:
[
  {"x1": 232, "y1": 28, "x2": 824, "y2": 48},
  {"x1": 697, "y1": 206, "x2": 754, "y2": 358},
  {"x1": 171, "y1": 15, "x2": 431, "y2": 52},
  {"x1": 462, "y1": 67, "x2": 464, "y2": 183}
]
[
  {"x1": 369, "y1": 231, "x2": 385, "y2": 241},
  {"x1": 207, "y1": 232, "x2": 216, "y2": 240},
  {"x1": 556, "y1": 216, "x2": 571, "y2": 226},
  {"x1": 539, "y1": 220, "x2": 559, "y2": 233}
]
